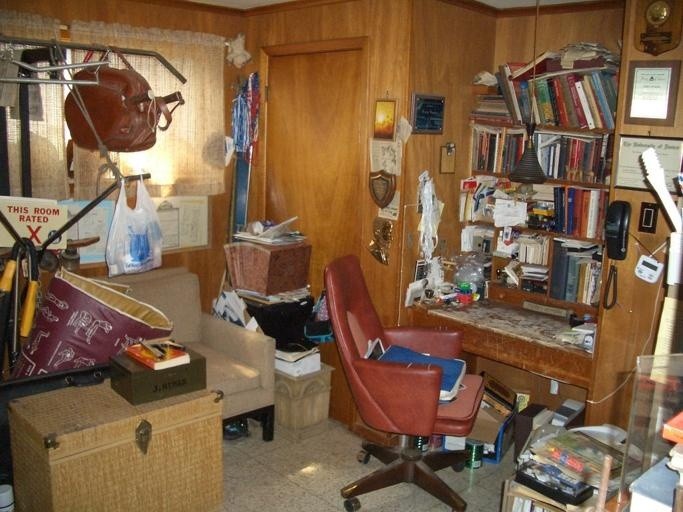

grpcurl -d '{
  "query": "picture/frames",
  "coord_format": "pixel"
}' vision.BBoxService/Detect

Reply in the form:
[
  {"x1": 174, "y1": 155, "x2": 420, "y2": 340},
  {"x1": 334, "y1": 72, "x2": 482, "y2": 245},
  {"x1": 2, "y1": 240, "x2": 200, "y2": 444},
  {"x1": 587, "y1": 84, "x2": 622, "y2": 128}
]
[
  {"x1": 440, "y1": 145, "x2": 456, "y2": 174},
  {"x1": 614, "y1": 134, "x2": 683, "y2": 197},
  {"x1": 410, "y1": 92, "x2": 444, "y2": 133},
  {"x1": 371, "y1": 100, "x2": 399, "y2": 142},
  {"x1": 625, "y1": 60, "x2": 682, "y2": 127}
]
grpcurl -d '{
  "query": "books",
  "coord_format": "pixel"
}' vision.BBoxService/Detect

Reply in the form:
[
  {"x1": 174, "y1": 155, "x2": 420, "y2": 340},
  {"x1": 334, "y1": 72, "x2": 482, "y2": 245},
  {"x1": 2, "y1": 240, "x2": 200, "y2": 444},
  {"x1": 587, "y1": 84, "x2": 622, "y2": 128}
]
[
  {"x1": 662, "y1": 410, "x2": 683, "y2": 487},
  {"x1": 471, "y1": 125, "x2": 615, "y2": 241},
  {"x1": 124, "y1": 338, "x2": 191, "y2": 370},
  {"x1": 471, "y1": 225, "x2": 605, "y2": 306},
  {"x1": 228, "y1": 214, "x2": 326, "y2": 381},
  {"x1": 470, "y1": 39, "x2": 619, "y2": 132}
]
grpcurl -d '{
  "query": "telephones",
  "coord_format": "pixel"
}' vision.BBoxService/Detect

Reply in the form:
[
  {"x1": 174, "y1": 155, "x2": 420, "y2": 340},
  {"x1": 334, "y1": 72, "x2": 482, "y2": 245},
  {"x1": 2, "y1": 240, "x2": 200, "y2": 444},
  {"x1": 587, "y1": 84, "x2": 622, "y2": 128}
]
[{"x1": 605, "y1": 201, "x2": 631, "y2": 261}]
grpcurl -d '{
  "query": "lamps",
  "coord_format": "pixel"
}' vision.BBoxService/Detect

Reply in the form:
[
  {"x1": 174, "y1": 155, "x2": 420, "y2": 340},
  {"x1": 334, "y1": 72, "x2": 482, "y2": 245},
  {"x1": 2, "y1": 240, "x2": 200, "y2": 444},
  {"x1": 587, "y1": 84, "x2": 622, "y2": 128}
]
[
  {"x1": 507, "y1": 0, "x2": 547, "y2": 184},
  {"x1": 645, "y1": 1, "x2": 668, "y2": 26}
]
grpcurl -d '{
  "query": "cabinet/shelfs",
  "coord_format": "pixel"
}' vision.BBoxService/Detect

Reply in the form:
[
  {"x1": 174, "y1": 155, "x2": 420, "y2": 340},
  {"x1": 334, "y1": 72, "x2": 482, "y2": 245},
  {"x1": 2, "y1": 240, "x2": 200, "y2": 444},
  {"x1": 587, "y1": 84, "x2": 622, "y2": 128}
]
[{"x1": 461, "y1": 115, "x2": 615, "y2": 321}]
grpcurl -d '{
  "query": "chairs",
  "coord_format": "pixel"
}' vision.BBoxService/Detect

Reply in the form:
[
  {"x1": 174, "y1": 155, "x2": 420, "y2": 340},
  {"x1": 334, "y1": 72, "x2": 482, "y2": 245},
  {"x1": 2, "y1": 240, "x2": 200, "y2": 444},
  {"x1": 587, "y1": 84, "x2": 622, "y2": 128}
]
[
  {"x1": 108, "y1": 268, "x2": 275, "y2": 446},
  {"x1": 325, "y1": 255, "x2": 484, "y2": 511}
]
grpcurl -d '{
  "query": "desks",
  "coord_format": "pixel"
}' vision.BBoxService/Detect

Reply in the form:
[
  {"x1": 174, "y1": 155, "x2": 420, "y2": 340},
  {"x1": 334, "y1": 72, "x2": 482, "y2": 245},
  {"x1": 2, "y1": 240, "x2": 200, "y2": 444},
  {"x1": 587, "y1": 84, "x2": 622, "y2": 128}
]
[{"x1": 411, "y1": 280, "x2": 599, "y2": 426}]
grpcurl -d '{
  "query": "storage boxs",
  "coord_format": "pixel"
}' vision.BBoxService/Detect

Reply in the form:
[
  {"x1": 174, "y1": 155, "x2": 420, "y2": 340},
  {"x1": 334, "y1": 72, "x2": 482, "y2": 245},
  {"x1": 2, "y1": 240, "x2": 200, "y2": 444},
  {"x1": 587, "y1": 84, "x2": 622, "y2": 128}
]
[
  {"x1": 224, "y1": 242, "x2": 310, "y2": 294},
  {"x1": 110, "y1": 347, "x2": 208, "y2": 406},
  {"x1": 275, "y1": 364, "x2": 334, "y2": 429},
  {"x1": 12, "y1": 385, "x2": 223, "y2": 512},
  {"x1": 0, "y1": 364, "x2": 109, "y2": 485},
  {"x1": 229, "y1": 295, "x2": 315, "y2": 345}
]
